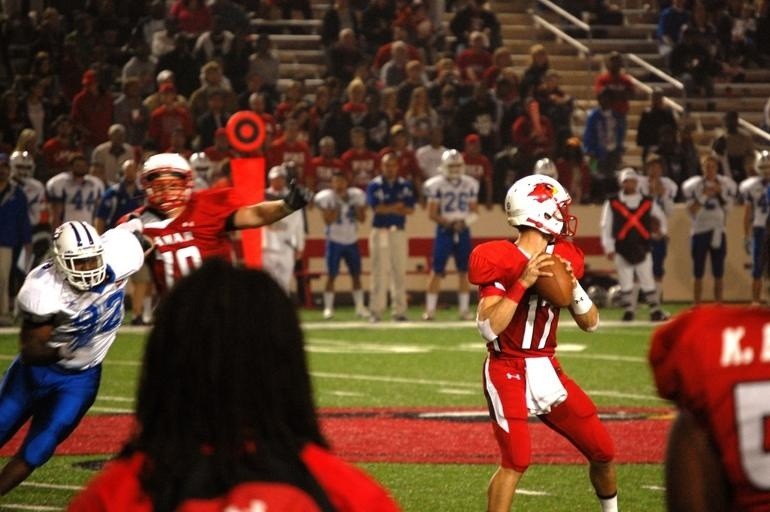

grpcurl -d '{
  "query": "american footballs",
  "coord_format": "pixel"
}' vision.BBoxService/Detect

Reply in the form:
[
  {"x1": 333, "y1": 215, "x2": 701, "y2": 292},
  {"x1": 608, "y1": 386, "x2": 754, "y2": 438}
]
[{"x1": 537, "y1": 251, "x2": 573, "y2": 307}]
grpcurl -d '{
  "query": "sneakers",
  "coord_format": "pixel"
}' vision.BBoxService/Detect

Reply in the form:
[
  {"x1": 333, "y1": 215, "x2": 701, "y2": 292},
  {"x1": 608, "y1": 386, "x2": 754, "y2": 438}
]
[
  {"x1": 324, "y1": 308, "x2": 474, "y2": 322},
  {"x1": 624, "y1": 309, "x2": 669, "y2": 321}
]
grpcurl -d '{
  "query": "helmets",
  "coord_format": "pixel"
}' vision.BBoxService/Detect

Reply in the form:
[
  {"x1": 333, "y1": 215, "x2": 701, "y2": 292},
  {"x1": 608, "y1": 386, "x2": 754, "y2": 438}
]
[
  {"x1": 11, "y1": 150, "x2": 36, "y2": 175},
  {"x1": 141, "y1": 152, "x2": 194, "y2": 211},
  {"x1": 534, "y1": 157, "x2": 558, "y2": 179},
  {"x1": 505, "y1": 174, "x2": 577, "y2": 244},
  {"x1": 441, "y1": 150, "x2": 466, "y2": 178},
  {"x1": 755, "y1": 152, "x2": 769, "y2": 175},
  {"x1": 52, "y1": 220, "x2": 107, "y2": 289}
]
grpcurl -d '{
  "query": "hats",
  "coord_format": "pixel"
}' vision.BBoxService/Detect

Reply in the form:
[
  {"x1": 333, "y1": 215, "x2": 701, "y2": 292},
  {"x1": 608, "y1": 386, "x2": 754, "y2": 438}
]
[
  {"x1": 619, "y1": 168, "x2": 640, "y2": 183},
  {"x1": 268, "y1": 165, "x2": 288, "y2": 179}
]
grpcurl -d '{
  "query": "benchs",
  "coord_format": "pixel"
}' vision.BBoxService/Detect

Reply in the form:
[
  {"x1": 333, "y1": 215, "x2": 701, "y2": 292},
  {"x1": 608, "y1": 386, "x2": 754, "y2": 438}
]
[
  {"x1": 441, "y1": 255, "x2": 619, "y2": 311},
  {"x1": 302, "y1": 256, "x2": 436, "y2": 312}
]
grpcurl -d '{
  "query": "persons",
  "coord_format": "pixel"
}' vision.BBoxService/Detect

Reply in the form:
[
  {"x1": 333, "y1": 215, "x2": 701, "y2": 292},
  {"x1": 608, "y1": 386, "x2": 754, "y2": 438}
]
[
  {"x1": 0, "y1": 219, "x2": 154, "y2": 498},
  {"x1": 468, "y1": 173, "x2": 618, "y2": 512},
  {"x1": 62, "y1": 258, "x2": 401, "y2": 512},
  {"x1": 1, "y1": 0, "x2": 769, "y2": 323},
  {"x1": 650, "y1": 301, "x2": 770, "y2": 511}
]
